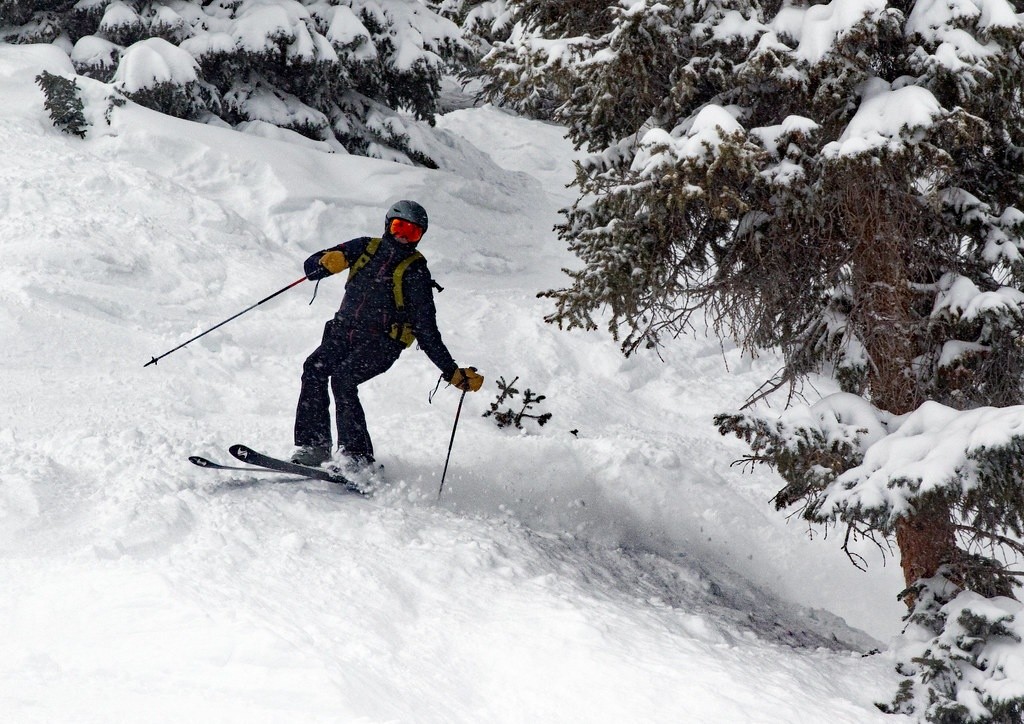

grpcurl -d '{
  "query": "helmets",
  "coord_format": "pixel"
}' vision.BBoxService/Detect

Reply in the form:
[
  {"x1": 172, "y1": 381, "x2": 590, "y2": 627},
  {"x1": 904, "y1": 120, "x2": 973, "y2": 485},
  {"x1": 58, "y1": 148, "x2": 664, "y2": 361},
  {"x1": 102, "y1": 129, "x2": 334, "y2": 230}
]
[{"x1": 384, "y1": 199, "x2": 428, "y2": 250}]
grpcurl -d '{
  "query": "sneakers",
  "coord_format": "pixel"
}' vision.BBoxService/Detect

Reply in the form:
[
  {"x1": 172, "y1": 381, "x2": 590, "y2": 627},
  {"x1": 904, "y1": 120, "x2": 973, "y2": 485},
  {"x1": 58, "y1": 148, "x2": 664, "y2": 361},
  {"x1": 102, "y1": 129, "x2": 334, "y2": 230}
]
[
  {"x1": 281, "y1": 444, "x2": 333, "y2": 467},
  {"x1": 324, "y1": 450, "x2": 375, "y2": 479}
]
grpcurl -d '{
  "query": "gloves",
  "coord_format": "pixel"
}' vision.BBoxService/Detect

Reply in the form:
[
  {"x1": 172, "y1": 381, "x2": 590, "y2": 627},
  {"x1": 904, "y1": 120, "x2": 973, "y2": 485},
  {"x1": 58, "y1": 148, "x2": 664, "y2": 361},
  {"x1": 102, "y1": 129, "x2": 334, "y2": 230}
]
[
  {"x1": 450, "y1": 366, "x2": 484, "y2": 392},
  {"x1": 315, "y1": 249, "x2": 350, "y2": 274}
]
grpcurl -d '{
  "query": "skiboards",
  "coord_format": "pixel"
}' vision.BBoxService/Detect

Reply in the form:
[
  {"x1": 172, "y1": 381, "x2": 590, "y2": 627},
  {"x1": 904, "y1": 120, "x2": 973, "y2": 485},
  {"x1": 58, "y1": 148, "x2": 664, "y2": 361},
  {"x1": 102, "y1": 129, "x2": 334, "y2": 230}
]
[{"x1": 188, "y1": 444, "x2": 385, "y2": 492}]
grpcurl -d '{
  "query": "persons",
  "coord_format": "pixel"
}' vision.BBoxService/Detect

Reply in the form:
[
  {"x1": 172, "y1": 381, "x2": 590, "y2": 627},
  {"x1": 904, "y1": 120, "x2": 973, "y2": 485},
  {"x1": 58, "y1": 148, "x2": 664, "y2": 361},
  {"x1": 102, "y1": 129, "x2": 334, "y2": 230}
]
[{"x1": 293, "y1": 202, "x2": 484, "y2": 478}]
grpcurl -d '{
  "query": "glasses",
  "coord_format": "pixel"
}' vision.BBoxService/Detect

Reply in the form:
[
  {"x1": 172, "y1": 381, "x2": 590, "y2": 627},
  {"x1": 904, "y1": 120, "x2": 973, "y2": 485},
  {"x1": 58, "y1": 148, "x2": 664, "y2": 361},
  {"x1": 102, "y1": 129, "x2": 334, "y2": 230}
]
[{"x1": 389, "y1": 219, "x2": 423, "y2": 244}]
